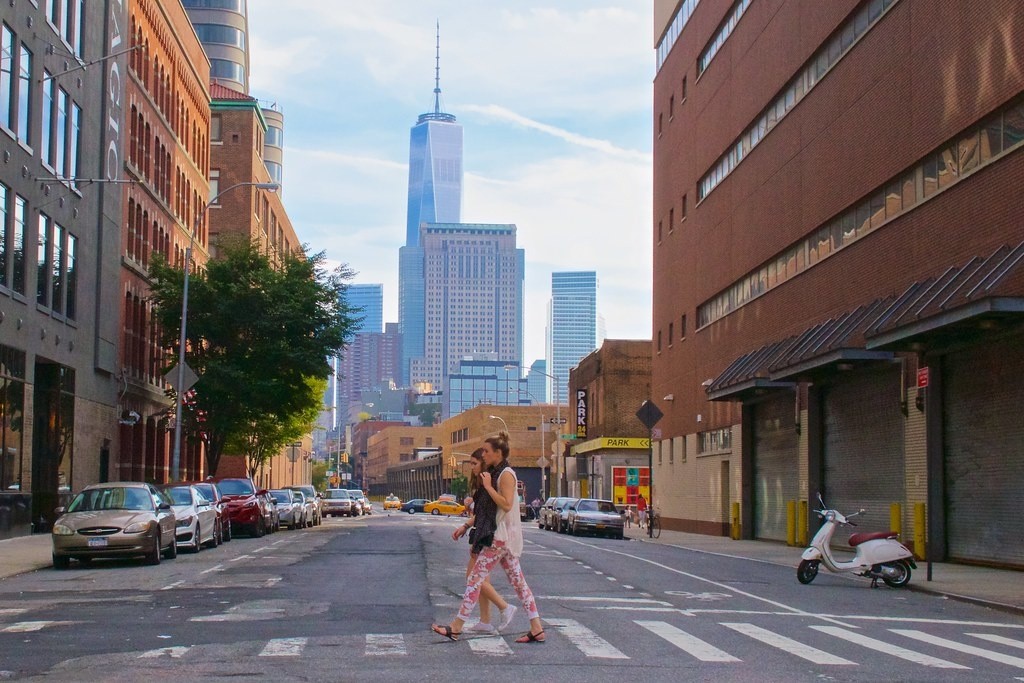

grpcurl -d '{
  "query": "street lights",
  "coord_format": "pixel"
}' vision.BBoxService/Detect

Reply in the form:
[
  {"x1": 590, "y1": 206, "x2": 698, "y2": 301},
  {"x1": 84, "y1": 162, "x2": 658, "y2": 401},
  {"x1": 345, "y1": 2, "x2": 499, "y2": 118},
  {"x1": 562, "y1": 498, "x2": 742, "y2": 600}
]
[
  {"x1": 168, "y1": 180, "x2": 278, "y2": 480},
  {"x1": 337, "y1": 402, "x2": 376, "y2": 488},
  {"x1": 503, "y1": 363, "x2": 566, "y2": 501},
  {"x1": 489, "y1": 414, "x2": 509, "y2": 432}
]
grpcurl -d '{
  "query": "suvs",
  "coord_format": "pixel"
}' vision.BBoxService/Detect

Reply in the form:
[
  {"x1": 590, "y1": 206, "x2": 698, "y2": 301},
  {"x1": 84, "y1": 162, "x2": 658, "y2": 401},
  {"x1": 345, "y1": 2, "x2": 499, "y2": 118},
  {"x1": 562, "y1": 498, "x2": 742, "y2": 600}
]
[
  {"x1": 320, "y1": 489, "x2": 365, "y2": 517},
  {"x1": 282, "y1": 484, "x2": 322, "y2": 526},
  {"x1": 212, "y1": 476, "x2": 267, "y2": 536}
]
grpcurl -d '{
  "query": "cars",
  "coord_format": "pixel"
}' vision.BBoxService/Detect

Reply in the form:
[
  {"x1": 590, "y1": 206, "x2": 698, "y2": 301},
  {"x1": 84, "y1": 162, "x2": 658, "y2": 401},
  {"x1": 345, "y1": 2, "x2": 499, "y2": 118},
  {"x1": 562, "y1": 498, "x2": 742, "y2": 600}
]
[
  {"x1": 383, "y1": 493, "x2": 400, "y2": 510},
  {"x1": 422, "y1": 497, "x2": 465, "y2": 516},
  {"x1": 51, "y1": 481, "x2": 175, "y2": 569},
  {"x1": 154, "y1": 482, "x2": 231, "y2": 552},
  {"x1": 538, "y1": 496, "x2": 625, "y2": 539},
  {"x1": 365, "y1": 498, "x2": 372, "y2": 514},
  {"x1": 255, "y1": 488, "x2": 320, "y2": 530},
  {"x1": 400, "y1": 499, "x2": 431, "y2": 514},
  {"x1": 0, "y1": 490, "x2": 75, "y2": 537}
]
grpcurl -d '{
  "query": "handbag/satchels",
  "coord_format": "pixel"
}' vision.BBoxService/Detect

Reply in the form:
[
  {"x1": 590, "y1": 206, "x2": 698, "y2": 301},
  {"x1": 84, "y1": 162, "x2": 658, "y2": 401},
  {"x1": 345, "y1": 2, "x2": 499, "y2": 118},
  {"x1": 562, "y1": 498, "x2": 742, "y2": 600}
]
[{"x1": 634, "y1": 516, "x2": 640, "y2": 524}]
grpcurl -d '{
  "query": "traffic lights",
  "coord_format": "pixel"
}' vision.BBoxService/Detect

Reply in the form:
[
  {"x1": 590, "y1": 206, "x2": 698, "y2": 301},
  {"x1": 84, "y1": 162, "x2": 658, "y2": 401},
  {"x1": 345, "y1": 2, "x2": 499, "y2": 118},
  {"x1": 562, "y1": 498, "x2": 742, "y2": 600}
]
[
  {"x1": 447, "y1": 457, "x2": 452, "y2": 466},
  {"x1": 454, "y1": 457, "x2": 456, "y2": 466},
  {"x1": 329, "y1": 477, "x2": 341, "y2": 483},
  {"x1": 340, "y1": 453, "x2": 349, "y2": 463}
]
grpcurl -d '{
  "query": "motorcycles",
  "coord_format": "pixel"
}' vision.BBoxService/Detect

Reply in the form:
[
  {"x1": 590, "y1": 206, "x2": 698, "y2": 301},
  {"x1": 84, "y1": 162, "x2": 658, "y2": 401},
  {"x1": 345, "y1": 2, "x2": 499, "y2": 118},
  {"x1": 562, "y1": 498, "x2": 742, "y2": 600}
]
[{"x1": 798, "y1": 493, "x2": 919, "y2": 590}]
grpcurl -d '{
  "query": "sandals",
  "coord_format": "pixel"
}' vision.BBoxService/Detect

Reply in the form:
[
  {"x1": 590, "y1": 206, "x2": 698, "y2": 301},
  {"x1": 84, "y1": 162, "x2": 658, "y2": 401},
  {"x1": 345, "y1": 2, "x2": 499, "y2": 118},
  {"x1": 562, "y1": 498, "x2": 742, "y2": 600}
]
[
  {"x1": 431, "y1": 624, "x2": 462, "y2": 641},
  {"x1": 515, "y1": 631, "x2": 545, "y2": 642}
]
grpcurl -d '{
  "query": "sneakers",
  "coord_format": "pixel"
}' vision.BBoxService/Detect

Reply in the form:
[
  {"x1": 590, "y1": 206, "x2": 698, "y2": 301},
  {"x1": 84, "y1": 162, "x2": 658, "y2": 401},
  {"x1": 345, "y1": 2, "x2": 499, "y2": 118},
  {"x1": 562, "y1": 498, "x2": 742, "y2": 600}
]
[
  {"x1": 498, "y1": 604, "x2": 516, "y2": 631},
  {"x1": 468, "y1": 622, "x2": 495, "y2": 633}
]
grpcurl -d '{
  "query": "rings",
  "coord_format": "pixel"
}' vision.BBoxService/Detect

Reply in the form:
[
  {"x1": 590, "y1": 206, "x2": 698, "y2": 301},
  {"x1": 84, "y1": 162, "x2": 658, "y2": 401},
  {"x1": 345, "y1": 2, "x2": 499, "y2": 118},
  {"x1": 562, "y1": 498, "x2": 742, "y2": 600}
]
[{"x1": 466, "y1": 502, "x2": 469, "y2": 504}]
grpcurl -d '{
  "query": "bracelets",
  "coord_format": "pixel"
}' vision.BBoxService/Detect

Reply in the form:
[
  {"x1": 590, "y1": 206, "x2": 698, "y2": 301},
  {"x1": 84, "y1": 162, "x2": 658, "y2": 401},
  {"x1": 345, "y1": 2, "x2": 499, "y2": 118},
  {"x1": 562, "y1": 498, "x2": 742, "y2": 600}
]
[{"x1": 463, "y1": 522, "x2": 470, "y2": 529}]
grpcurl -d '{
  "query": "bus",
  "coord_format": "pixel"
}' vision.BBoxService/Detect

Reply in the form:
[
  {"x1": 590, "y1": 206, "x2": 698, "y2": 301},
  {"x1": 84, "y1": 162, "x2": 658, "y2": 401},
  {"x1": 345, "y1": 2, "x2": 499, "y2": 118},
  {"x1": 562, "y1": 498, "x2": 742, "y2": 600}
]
[{"x1": 517, "y1": 480, "x2": 527, "y2": 522}]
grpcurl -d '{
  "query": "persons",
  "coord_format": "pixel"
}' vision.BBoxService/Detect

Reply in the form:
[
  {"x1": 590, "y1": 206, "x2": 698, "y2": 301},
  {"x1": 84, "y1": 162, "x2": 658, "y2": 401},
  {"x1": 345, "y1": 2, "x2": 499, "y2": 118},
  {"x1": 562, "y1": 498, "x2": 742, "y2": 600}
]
[
  {"x1": 624, "y1": 505, "x2": 637, "y2": 528},
  {"x1": 637, "y1": 494, "x2": 647, "y2": 529},
  {"x1": 531, "y1": 497, "x2": 545, "y2": 519},
  {"x1": 430, "y1": 431, "x2": 547, "y2": 643}
]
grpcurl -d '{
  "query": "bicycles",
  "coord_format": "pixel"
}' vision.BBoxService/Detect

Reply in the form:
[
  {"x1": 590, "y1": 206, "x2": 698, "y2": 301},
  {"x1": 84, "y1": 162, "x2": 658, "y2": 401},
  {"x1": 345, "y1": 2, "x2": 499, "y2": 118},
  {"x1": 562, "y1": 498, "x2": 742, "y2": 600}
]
[{"x1": 643, "y1": 507, "x2": 663, "y2": 537}]
